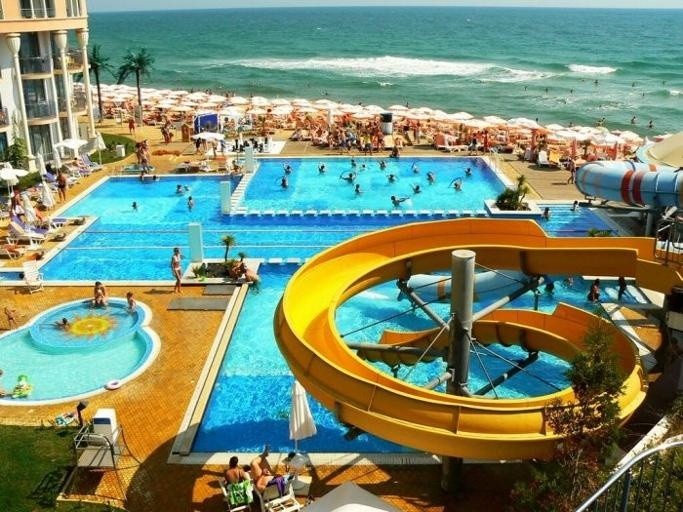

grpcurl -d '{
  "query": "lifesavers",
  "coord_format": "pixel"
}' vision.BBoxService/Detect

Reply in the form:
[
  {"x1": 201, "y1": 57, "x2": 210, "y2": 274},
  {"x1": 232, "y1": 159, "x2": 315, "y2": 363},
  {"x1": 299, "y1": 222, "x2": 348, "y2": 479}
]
[{"x1": 106, "y1": 380, "x2": 121, "y2": 390}]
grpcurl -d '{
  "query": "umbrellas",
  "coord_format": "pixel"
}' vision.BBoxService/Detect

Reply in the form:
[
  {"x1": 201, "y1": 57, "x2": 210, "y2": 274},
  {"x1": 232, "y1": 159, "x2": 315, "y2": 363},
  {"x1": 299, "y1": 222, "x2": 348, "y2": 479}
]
[
  {"x1": 50, "y1": 144, "x2": 61, "y2": 169},
  {"x1": 34, "y1": 153, "x2": 47, "y2": 176},
  {"x1": 288, "y1": 379, "x2": 319, "y2": 455},
  {"x1": 53, "y1": 137, "x2": 88, "y2": 152},
  {"x1": 39, "y1": 180, "x2": 56, "y2": 215},
  {"x1": 0, "y1": 161, "x2": 29, "y2": 197}
]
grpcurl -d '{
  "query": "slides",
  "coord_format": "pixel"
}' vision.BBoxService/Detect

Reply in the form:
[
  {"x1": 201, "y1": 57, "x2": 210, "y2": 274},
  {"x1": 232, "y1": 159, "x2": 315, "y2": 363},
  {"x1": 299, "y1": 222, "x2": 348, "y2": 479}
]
[
  {"x1": 575, "y1": 130, "x2": 683, "y2": 206},
  {"x1": 273, "y1": 216, "x2": 683, "y2": 460}
]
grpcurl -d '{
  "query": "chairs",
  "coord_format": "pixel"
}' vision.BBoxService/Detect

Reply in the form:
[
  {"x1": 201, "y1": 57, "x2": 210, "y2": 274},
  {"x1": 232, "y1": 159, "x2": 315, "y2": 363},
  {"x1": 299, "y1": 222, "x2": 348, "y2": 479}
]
[
  {"x1": 0, "y1": 152, "x2": 103, "y2": 260},
  {"x1": 217, "y1": 478, "x2": 305, "y2": 512},
  {"x1": 22, "y1": 260, "x2": 44, "y2": 294},
  {"x1": 538, "y1": 150, "x2": 550, "y2": 167}
]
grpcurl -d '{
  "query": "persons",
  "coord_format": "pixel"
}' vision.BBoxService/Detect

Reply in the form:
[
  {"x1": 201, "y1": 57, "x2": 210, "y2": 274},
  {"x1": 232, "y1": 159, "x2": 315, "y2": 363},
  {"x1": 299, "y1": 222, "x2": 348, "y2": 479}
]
[
  {"x1": 222, "y1": 455, "x2": 244, "y2": 486},
  {"x1": 540, "y1": 207, "x2": 551, "y2": 219},
  {"x1": 542, "y1": 274, "x2": 554, "y2": 296},
  {"x1": 69, "y1": 74, "x2": 666, "y2": 175},
  {"x1": 573, "y1": 201, "x2": 579, "y2": 212},
  {"x1": 242, "y1": 265, "x2": 261, "y2": 293},
  {"x1": 390, "y1": 195, "x2": 409, "y2": 208},
  {"x1": 463, "y1": 168, "x2": 472, "y2": 177},
  {"x1": 130, "y1": 201, "x2": 139, "y2": 214},
  {"x1": 617, "y1": 276, "x2": 626, "y2": 300},
  {"x1": 586, "y1": 278, "x2": 600, "y2": 302},
  {"x1": 186, "y1": 195, "x2": 194, "y2": 211},
  {"x1": 125, "y1": 291, "x2": 136, "y2": 314},
  {"x1": 453, "y1": 182, "x2": 462, "y2": 192},
  {"x1": 249, "y1": 444, "x2": 276, "y2": 482},
  {"x1": 183, "y1": 184, "x2": 190, "y2": 191},
  {"x1": 0, "y1": 369, "x2": 7, "y2": 398},
  {"x1": 140, "y1": 170, "x2": 144, "y2": 183},
  {"x1": 663, "y1": 337, "x2": 682, "y2": 366},
  {"x1": 386, "y1": 174, "x2": 400, "y2": 183},
  {"x1": 54, "y1": 169, "x2": 67, "y2": 203},
  {"x1": 150, "y1": 174, "x2": 159, "y2": 182},
  {"x1": 0, "y1": 189, "x2": 46, "y2": 262},
  {"x1": 170, "y1": 246, "x2": 183, "y2": 294},
  {"x1": 91, "y1": 282, "x2": 108, "y2": 307},
  {"x1": 339, "y1": 171, "x2": 357, "y2": 184},
  {"x1": 174, "y1": 184, "x2": 183, "y2": 194},
  {"x1": 280, "y1": 451, "x2": 295, "y2": 465},
  {"x1": 3, "y1": 305, "x2": 18, "y2": 330},
  {"x1": 54, "y1": 316, "x2": 69, "y2": 331},
  {"x1": 281, "y1": 176, "x2": 288, "y2": 189},
  {"x1": 567, "y1": 155, "x2": 577, "y2": 184},
  {"x1": 425, "y1": 172, "x2": 434, "y2": 185},
  {"x1": 354, "y1": 183, "x2": 362, "y2": 192},
  {"x1": 408, "y1": 183, "x2": 422, "y2": 193}
]
